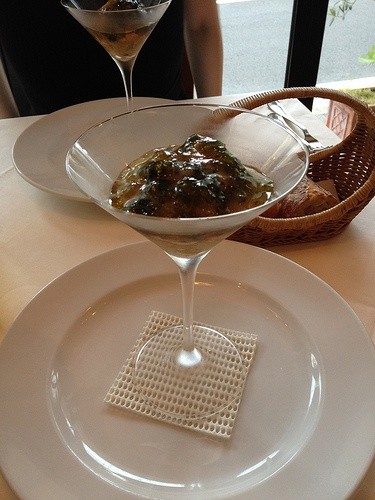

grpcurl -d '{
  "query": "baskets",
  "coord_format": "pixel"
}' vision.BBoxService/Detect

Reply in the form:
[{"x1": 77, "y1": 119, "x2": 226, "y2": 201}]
[{"x1": 192, "y1": 87, "x2": 375, "y2": 248}]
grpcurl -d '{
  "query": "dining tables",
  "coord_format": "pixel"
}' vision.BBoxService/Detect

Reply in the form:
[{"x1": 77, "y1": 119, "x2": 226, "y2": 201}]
[{"x1": 0, "y1": 77, "x2": 375, "y2": 500}]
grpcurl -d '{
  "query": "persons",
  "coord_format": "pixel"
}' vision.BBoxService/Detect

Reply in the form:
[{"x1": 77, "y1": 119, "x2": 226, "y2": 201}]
[{"x1": 0, "y1": 0, "x2": 223, "y2": 119}]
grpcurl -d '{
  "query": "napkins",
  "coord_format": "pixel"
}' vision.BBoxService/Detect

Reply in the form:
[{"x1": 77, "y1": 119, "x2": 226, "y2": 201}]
[{"x1": 262, "y1": 98, "x2": 343, "y2": 148}]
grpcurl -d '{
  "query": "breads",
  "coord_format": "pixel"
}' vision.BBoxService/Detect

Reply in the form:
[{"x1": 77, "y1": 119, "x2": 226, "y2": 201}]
[{"x1": 260, "y1": 172, "x2": 341, "y2": 218}]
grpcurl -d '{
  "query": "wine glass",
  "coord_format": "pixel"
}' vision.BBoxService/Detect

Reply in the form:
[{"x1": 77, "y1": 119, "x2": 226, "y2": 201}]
[
  {"x1": 64, "y1": 104, "x2": 309, "y2": 420},
  {"x1": 59, "y1": 0, "x2": 172, "y2": 110}
]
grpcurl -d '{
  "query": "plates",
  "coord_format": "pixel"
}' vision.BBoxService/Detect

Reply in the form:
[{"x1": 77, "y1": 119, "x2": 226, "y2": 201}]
[
  {"x1": 0, "y1": 239, "x2": 375, "y2": 500},
  {"x1": 11, "y1": 97, "x2": 180, "y2": 203}
]
